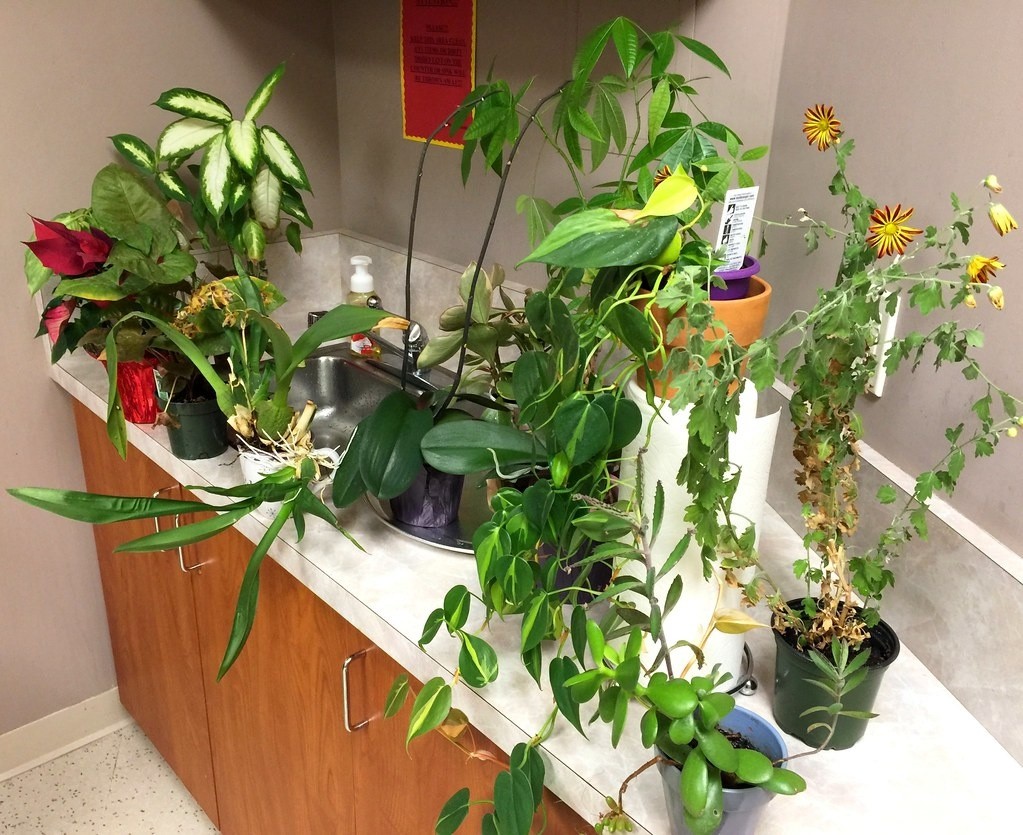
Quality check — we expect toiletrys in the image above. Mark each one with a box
[348,255,381,357]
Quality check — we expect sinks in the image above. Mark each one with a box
[225,328,458,487]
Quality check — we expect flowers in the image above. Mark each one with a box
[653,99,1023,656]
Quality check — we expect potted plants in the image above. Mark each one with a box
[7,19,805,835]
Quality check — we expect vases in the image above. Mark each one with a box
[632,276,773,403]
[767,599,901,751]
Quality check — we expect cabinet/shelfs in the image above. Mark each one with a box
[71,401,356,835]
[345,622,597,835]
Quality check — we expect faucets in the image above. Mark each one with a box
[309,296,446,391]
